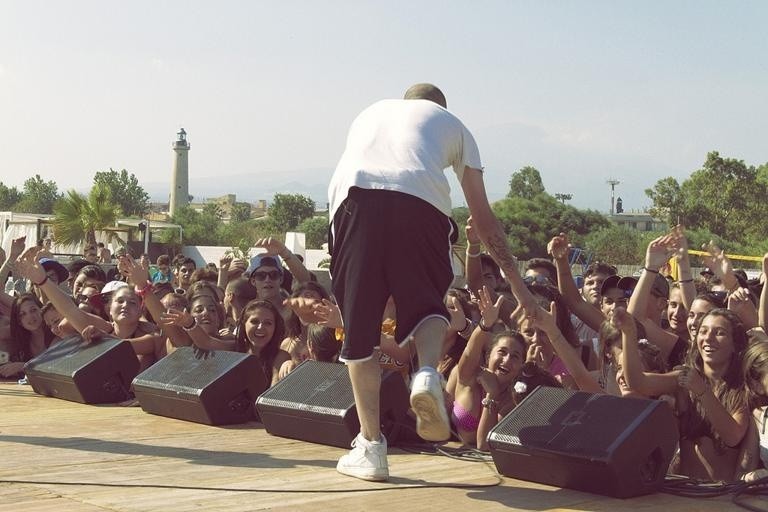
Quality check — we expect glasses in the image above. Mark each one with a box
[521,272,553,285]
[251,270,282,282]
[99,296,110,305]
[174,288,187,296]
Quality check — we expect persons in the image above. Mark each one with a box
[1,215,768,482]
[327,83,540,484]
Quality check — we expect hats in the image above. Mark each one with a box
[36,257,69,284]
[87,279,129,312]
[600,273,623,297]
[699,267,715,277]
[617,267,671,302]
[245,252,286,281]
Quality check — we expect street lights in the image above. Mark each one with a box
[606,179,620,214]
[139,218,150,257]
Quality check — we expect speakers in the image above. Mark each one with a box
[486,385,681,498]
[256,359,421,452]
[23,334,143,404]
[131,345,265,427]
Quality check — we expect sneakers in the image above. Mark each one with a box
[410,367,454,445]
[336,431,392,483]
